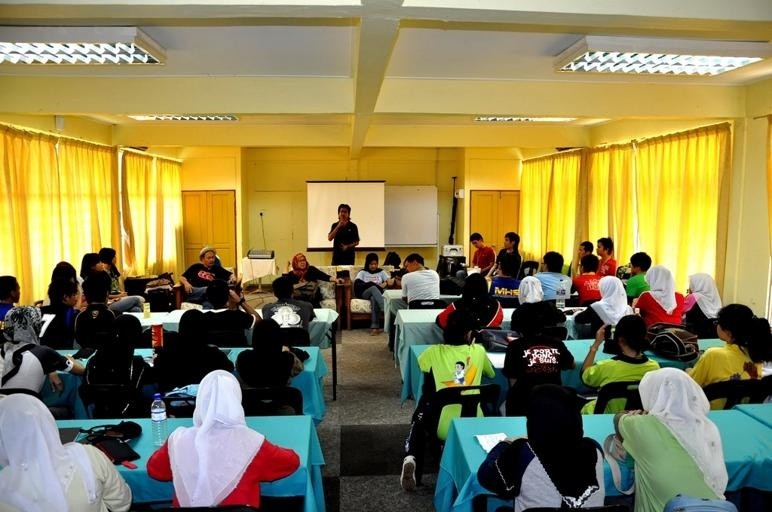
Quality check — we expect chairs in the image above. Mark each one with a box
[173,267,242,311]
[290,265,343,330]
[344,265,395,329]
[518,261,539,281]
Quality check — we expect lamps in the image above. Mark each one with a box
[1,25,167,67]
[553,36,766,79]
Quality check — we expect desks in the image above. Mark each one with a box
[243,257,275,293]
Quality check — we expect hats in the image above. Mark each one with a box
[200,247,215,260]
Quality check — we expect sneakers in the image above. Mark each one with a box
[400,455,416,490]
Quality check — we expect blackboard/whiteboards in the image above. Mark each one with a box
[384,184,440,247]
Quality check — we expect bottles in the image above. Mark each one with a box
[150,392,168,446]
[556,279,566,310]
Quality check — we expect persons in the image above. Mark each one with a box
[353,232,772,512]
[329,203,360,266]
[1,248,337,511]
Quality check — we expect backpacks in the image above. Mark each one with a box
[648,322,698,361]
